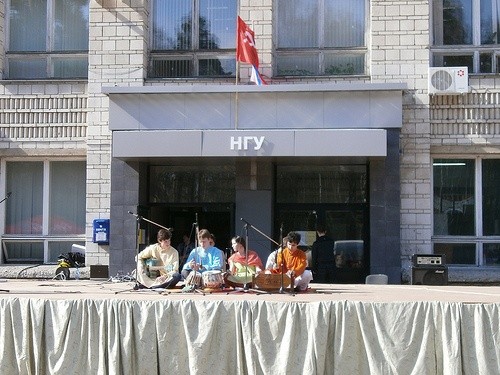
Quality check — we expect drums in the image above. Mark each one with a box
[202,270,223,287]
[254,273,291,288]
[186,270,202,287]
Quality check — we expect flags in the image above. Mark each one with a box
[236,16,269,87]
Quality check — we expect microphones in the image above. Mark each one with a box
[169,227,174,232]
[0,191,12,203]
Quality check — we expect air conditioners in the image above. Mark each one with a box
[428,66,471,95]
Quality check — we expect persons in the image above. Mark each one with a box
[208,234,226,274]
[269,233,311,292]
[325,243,359,284]
[312,222,334,284]
[265,236,288,275]
[176,233,195,274]
[181,230,224,288]
[130,229,181,290]
[224,234,264,289]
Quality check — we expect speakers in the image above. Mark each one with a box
[412,265,448,286]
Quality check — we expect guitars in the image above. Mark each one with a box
[144,258,173,278]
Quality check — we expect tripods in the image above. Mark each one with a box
[114,210,298,297]
